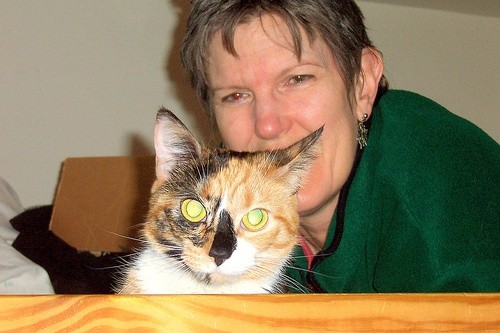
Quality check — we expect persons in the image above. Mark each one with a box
[179,1,499,295]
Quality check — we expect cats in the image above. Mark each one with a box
[110,107,326,295]
